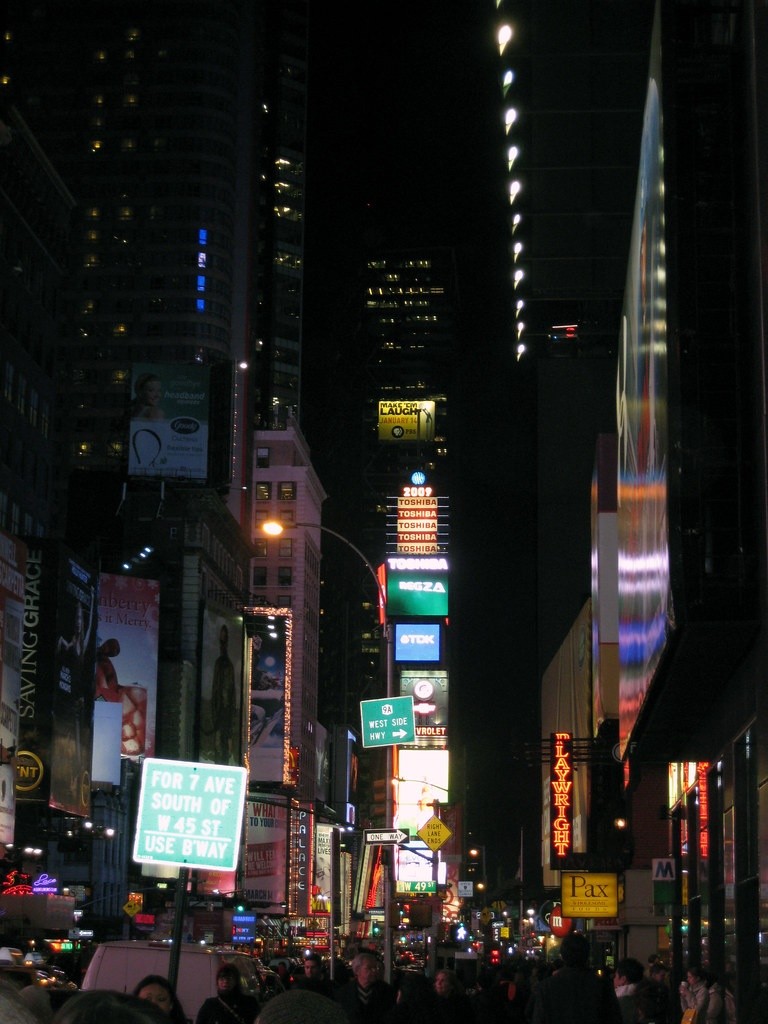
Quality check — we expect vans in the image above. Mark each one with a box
[82,942,279,1014]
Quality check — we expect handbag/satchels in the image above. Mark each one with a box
[682,1008,699,1024]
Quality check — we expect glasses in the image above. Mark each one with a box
[219,975,234,981]
[360,966,378,972]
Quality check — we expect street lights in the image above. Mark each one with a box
[397,776,467,883]
[263,521,394,989]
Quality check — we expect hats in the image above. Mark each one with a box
[258,990,348,1024]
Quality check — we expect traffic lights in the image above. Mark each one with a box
[450,925,471,947]
[372,923,379,937]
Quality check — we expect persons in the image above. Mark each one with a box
[133,974,187,1024]
[388,969,475,1024]
[132,373,164,418]
[211,625,236,764]
[52,989,170,1024]
[334,953,398,1024]
[292,954,334,1001]
[724,976,737,1024]
[515,933,623,1024]
[614,954,669,1024]
[678,968,723,1024]
[194,964,258,1024]
[0,975,52,1024]
[278,962,289,990]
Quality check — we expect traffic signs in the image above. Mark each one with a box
[360,695,419,750]
[362,829,410,845]
